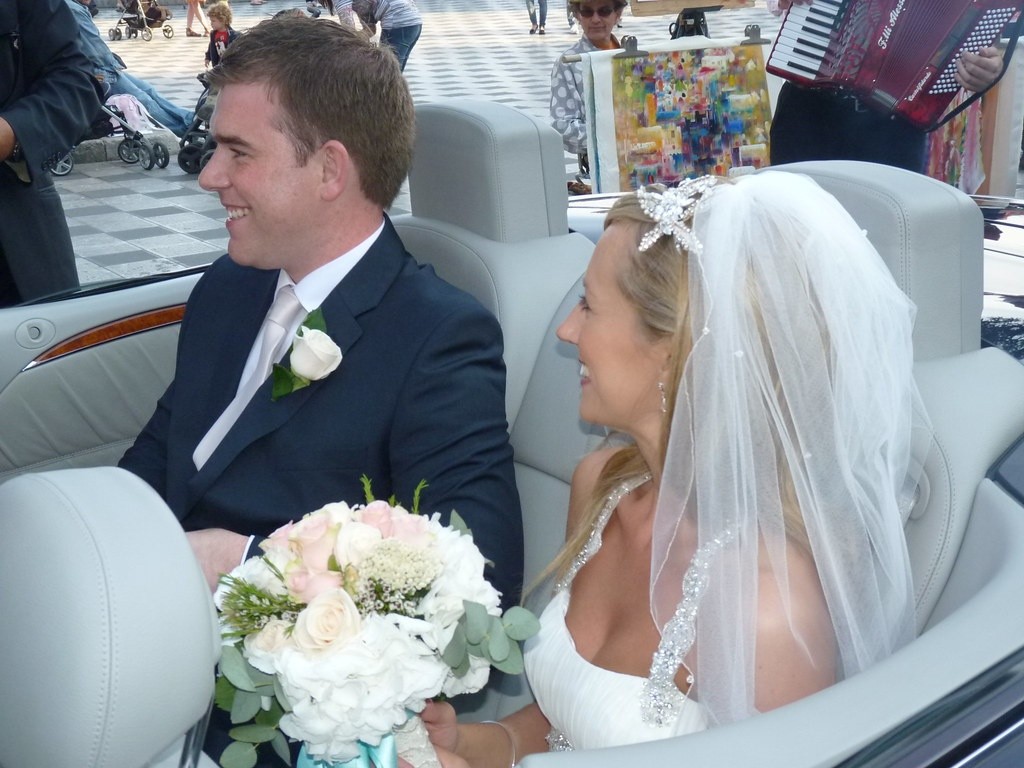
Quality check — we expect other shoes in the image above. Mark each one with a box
[186,28,200,36]
[539,25,544,34]
[530,25,538,34]
[204,31,209,37]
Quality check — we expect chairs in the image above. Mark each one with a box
[509,156,983,637]
[388,92,595,422]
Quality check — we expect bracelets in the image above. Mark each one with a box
[480,721,516,768]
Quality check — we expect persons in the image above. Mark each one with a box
[274,8,305,18]
[77,0,98,17]
[370,171,935,768]
[551,0,627,175]
[617,17,623,27]
[205,4,244,68]
[116,16,525,768]
[305,0,321,18]
[567,0,578,33]
[318,0,423,75]
[526,0,547,34]
[767,0,1004,174]
[0,0,104,309]
[185,0,210,36]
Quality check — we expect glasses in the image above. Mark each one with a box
[577,7,616,17]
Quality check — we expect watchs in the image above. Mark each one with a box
[7,140,24,163]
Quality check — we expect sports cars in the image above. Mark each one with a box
[0,95,1024,767]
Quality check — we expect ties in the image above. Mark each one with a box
[193,286,302,473]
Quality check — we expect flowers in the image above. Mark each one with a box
[214,475,541,768]
[268,309,344,400]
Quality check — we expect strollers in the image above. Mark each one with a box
[107,0,175,41]
[49,79,168,176]
[179,73,218,173]
